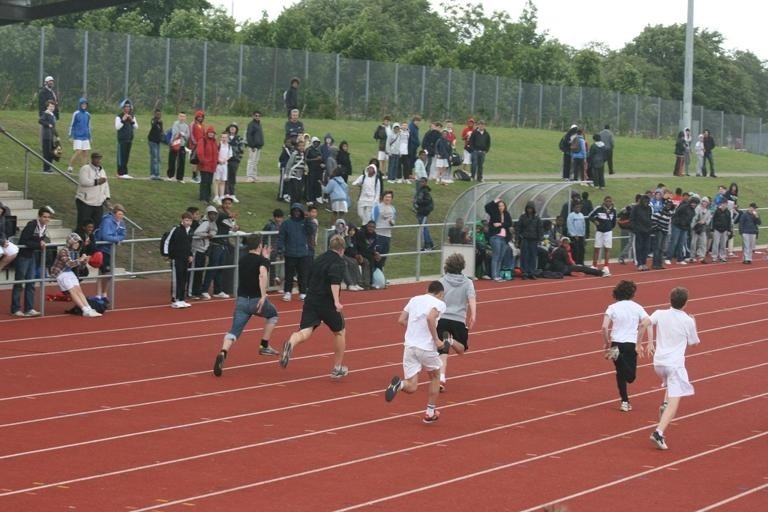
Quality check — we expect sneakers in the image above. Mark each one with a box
[382,175,484,186]
[618,251,752,272]
[168,277,391,308]
[283,195,329,208]
[563,176,606,190]
[605,346,667,452]
[42,166,261,183]
[201,194,240,206]
[13,296,111,317]
[214,332,454,423]
[673,173,718,178]
[473,264,611,281]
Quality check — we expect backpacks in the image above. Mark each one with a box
[570,137,581,153]
[558,133,570,151]
[617,205,635,230]
[160,231,170,256]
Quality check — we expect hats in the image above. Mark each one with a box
[45,76,55,82]
[206,205,218,214]
[432,118,486,133]
[750,202,758,208]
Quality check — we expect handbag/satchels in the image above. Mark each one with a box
[372,268,385,290]
[450,149,462,166]
[348,194,353,209]
[453,169,470,181]
[160,128,173,145]
[466,143,473,153]
[88,251,103,268]
[49,135,64,162]
[190,144,198,164]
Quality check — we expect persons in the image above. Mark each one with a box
[383,282,445,424]
[213,233,280,376]
[375,114,491,185]
[38,75,383,224]
[558,123,616,189]
[281,235,348,379]
[439,253,477,392]
[412,177,435,251]
[599,280,656,413]
[673,127,716,177]
[635,287,701,450]
[449,182,762,283]
[2,153,128,318]
[160,190,396,308]
